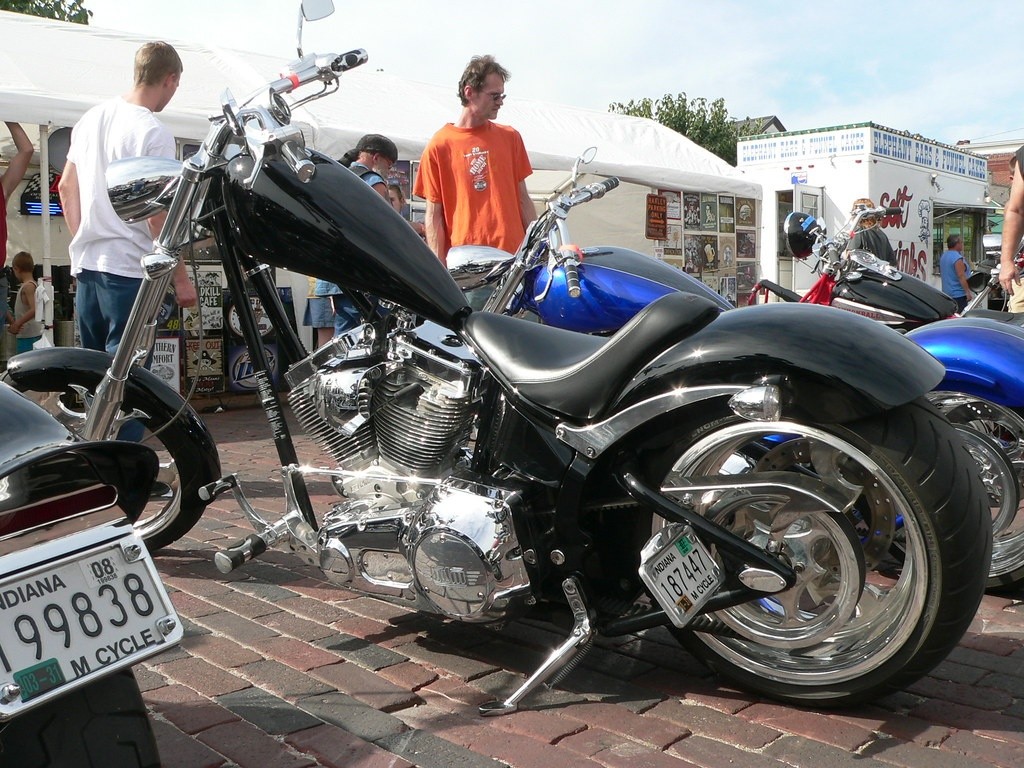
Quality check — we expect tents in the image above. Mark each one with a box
[0,8,764,349]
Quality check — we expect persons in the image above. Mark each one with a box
[846,199,898,270]
[0,41,425,498]
[998,146,1024,313]
[412,55,539,311]
[939,234,973,315]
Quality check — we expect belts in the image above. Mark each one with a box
[0,265,13,279]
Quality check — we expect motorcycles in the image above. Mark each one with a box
[1,0,1022,768]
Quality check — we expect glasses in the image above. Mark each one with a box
[480,87,507,101]
[382,155,393,170]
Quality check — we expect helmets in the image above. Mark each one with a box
[355,134,399,164]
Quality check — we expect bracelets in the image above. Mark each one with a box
[420,222,425,238]
